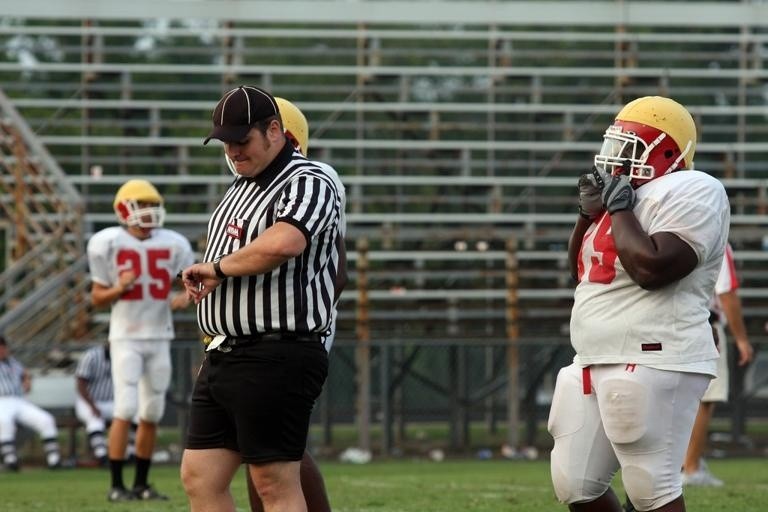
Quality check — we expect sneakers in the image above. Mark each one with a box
[102,479,167,503]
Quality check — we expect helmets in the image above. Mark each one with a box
[267,91,316,161]
[597,91,699,185]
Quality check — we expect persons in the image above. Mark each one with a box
[681,241,755,489]
[181,84,342,511]
[86,179,195,500]
[223,96,350,511]
[1,337,75,472]
[547,97,734,512]
[74,340,140,465]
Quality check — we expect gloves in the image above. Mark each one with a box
[592,163,635,218]
[571,166,606,220]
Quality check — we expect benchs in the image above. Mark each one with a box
[18,407,179,468]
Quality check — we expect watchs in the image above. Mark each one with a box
[212,252,231,280]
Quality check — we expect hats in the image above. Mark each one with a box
[197,83,277,149]
[113,179,167,234]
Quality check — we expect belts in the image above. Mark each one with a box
[219,327,324,349]
